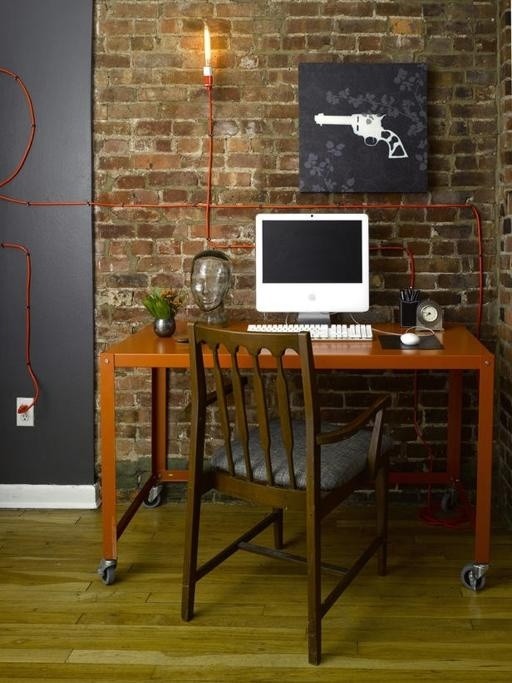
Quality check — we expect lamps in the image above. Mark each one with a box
[199,20,216,89]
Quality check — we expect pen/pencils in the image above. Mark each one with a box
[400,288,420,302]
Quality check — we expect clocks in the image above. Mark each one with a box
[414,298,445,332]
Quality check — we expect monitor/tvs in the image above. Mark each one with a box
[256,213,370,323]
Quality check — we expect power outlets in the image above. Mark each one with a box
[13,396,34,428]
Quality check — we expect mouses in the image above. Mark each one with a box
[400,333,420,346]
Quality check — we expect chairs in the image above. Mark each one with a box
[171,314,398,666]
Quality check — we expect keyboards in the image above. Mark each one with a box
[247,324,373,341]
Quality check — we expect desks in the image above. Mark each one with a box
[95,303,497,599]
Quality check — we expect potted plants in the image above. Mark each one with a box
[143,285,186,339]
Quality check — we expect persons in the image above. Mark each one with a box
[189,249,234,325]
